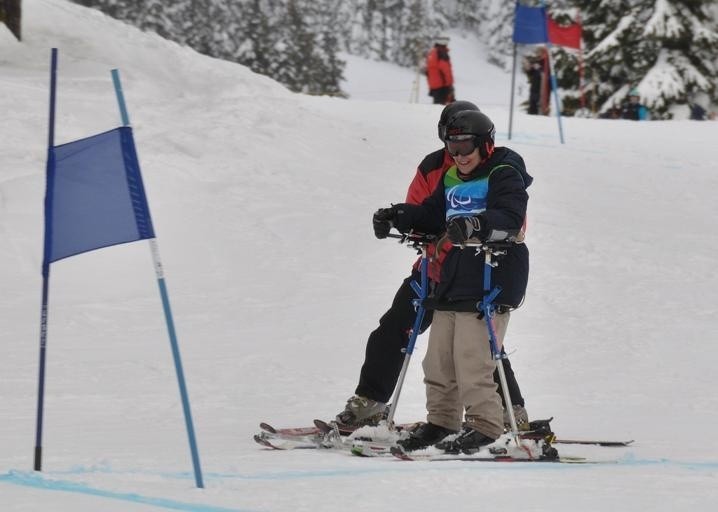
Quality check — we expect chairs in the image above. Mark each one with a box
[335,392,530,450]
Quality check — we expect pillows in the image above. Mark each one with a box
[438,101,496,159]
[435,30,450,48]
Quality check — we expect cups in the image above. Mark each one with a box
[446,127,496,157]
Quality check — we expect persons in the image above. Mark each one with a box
[337,101,529,432]
[427,36,456,106]
[373,110,534,454]
[523,45,552,116]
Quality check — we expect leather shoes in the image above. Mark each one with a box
[373,207,397,238]
[446,214,492,242]
